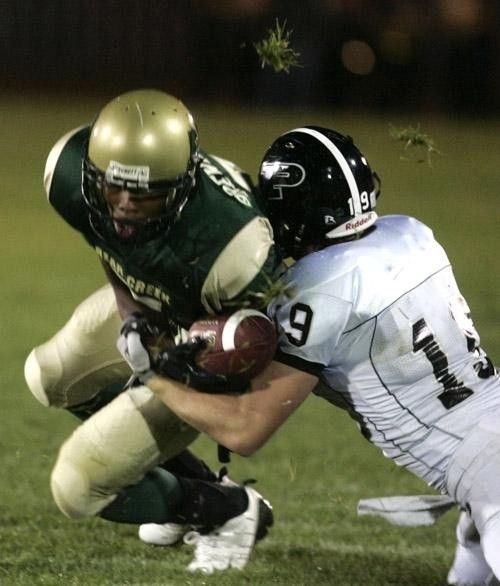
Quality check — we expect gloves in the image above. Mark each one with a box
[116,312,225,394]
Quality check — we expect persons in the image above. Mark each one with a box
[24,89,290,574]
[117,126,500,585]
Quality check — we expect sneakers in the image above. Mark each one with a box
[139,472,240,546]
[189,485,274,573]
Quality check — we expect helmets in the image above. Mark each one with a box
[259,126,381,258]
[82,89,199,247]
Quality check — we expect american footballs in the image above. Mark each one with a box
[173,308,281,382]
